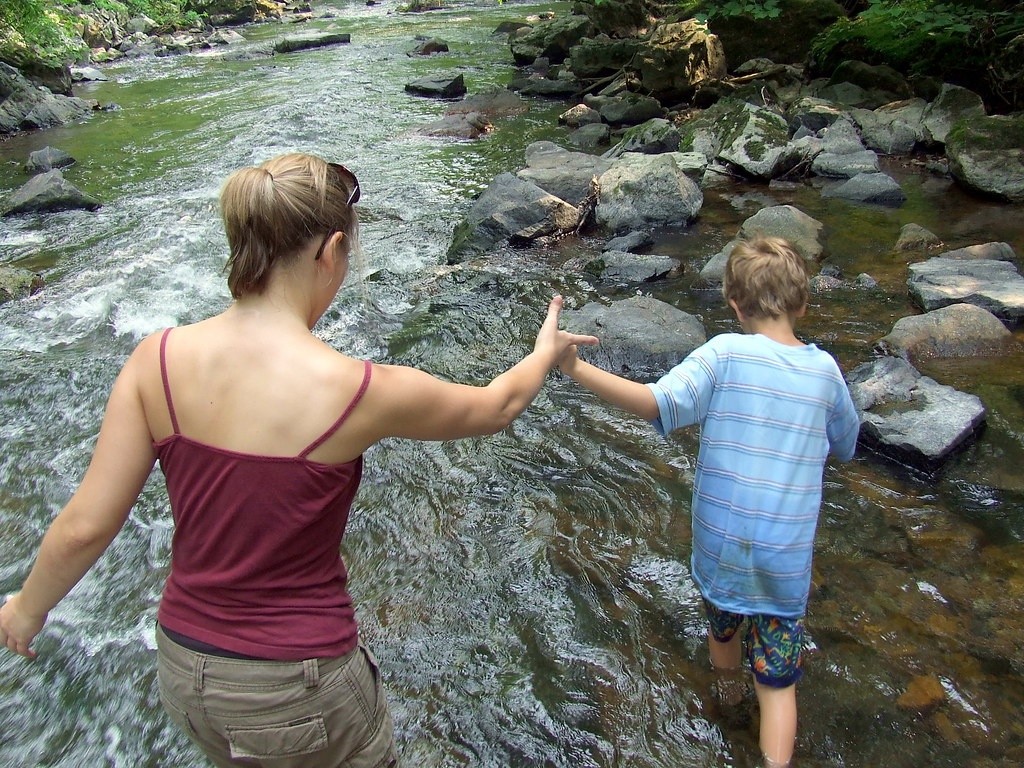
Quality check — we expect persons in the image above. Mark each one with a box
[1,154,598,768]
[555,235,858,768]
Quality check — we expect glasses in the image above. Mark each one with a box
[314,163,360,260]
